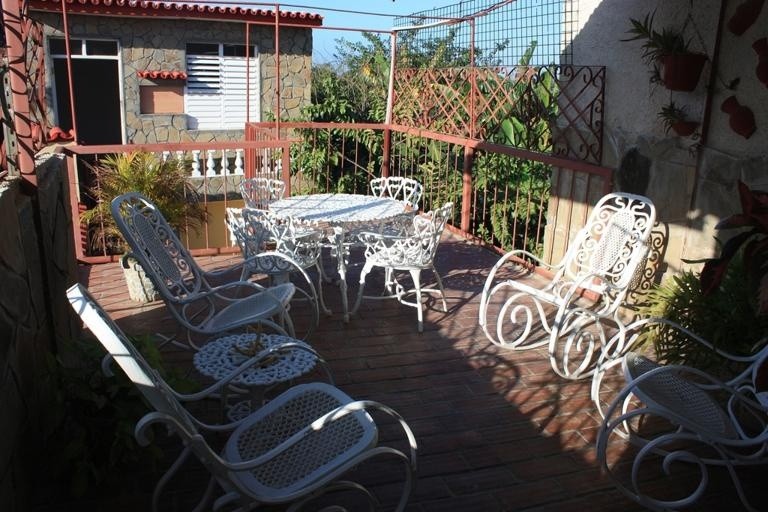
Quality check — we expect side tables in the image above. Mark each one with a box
[191,331,320,429]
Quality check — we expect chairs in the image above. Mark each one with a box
[224,206,333,325]
[370,176,426,208]
[108,191,321,352]
[589,316,768,510]
[352,200,457,334]
[477,190,658,382]
[64,278,422,511]
[238,177,287,211]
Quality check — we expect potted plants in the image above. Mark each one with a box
[655,100,703,141]
[617,3,709,94]
[78,146,214,304]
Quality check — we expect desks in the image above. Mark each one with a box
[267,192,420,322]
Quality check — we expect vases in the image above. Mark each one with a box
[727,0,765,36]
[751,37,768,90]
[720,95,758,140]
